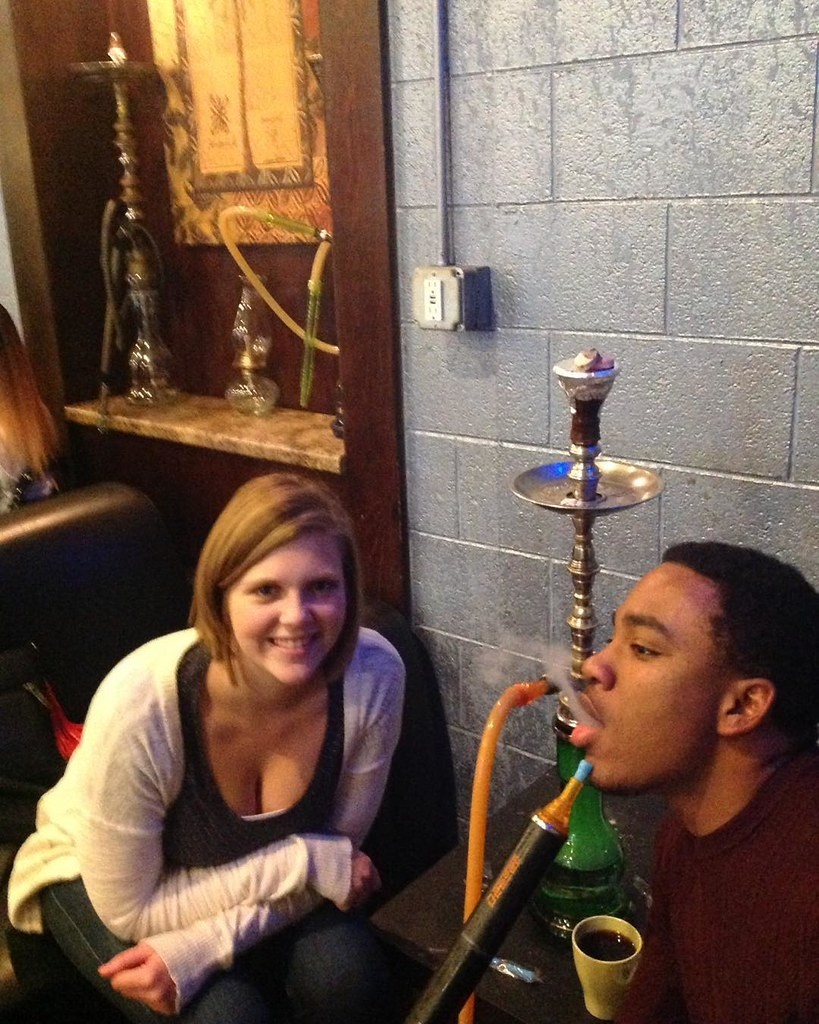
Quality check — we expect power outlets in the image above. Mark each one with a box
[410,267,475,332]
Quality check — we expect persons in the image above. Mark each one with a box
[0,302,103,513]
[570,541,819,1023]
[6,472,406,1023]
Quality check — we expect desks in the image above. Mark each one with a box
[369,766,670,1024]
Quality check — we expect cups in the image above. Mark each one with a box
[572,914,643,1020]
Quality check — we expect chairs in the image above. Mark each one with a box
[1,481,178,821]
[8,594,460,1024]
